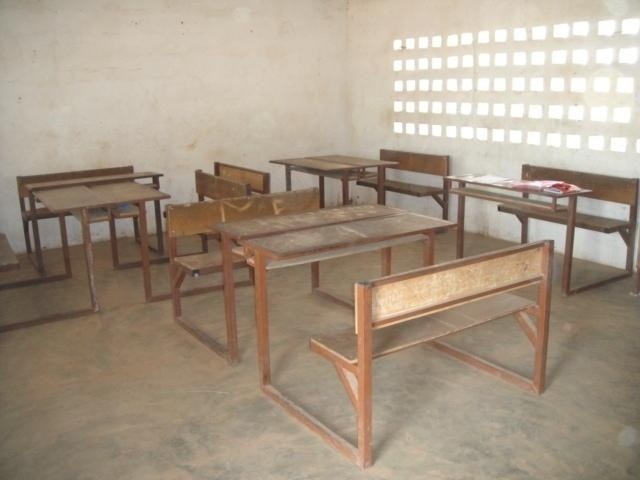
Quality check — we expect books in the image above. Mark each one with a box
[513,180,581,194]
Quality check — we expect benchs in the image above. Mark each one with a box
[1,150,639,469]
